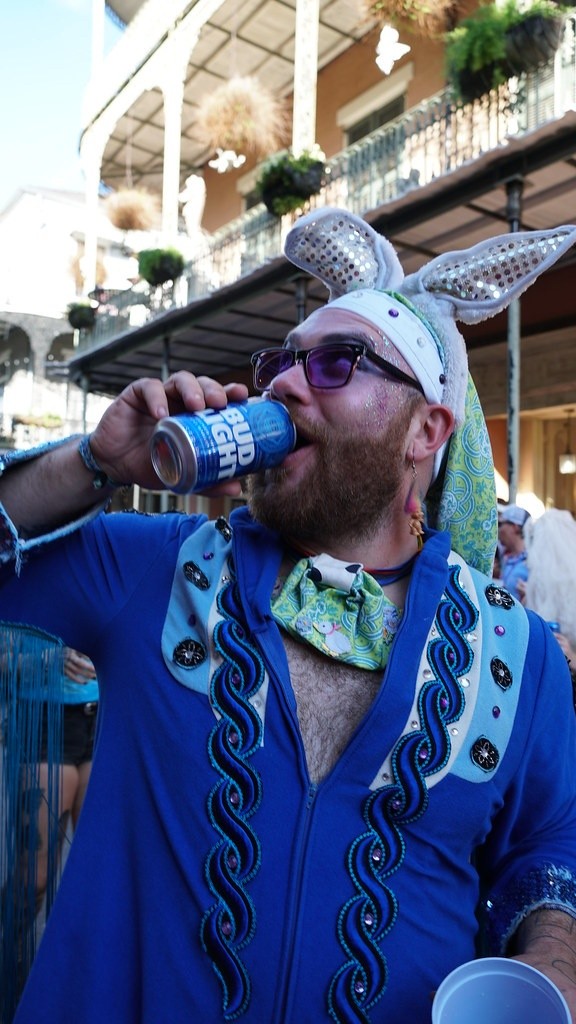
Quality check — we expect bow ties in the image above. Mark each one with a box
[269,536,420,673]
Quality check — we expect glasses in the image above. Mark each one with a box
[250,342,424,391]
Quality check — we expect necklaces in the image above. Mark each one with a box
[281,535,426,588]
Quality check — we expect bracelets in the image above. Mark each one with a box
[78,433,132,492]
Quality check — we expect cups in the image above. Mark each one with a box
[433,955,571,1024]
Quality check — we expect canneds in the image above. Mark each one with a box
[148,396,298,494]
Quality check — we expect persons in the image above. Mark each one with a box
[495,497,576,686]
[0,629,98,983]
[2,287,576,1023]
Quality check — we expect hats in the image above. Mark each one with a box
[498,508,531,526]
